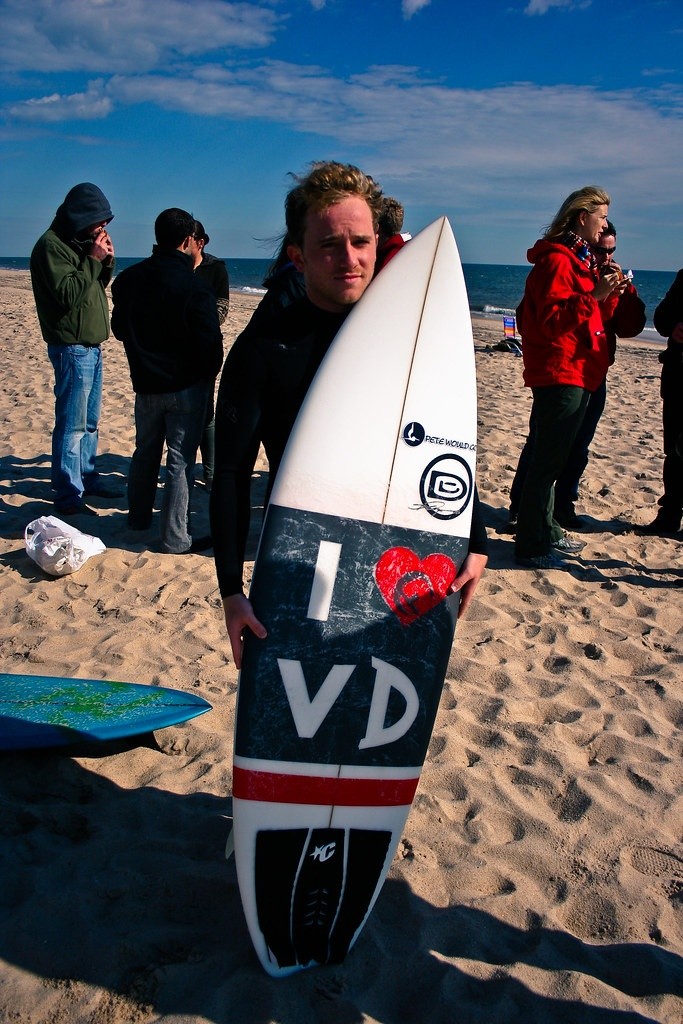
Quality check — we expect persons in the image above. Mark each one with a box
[207,163,489,671]
[646,268,683,536]
[30,183,124,516]
[111,207,229,554]
[506,186,646,570]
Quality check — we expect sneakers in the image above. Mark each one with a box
[204,476,213,492]
[525,531,589,569]
[506,511,521,528]
[85,482,124,499]
[552,502,584,529]
[55,500,98,517]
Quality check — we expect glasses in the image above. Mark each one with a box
[590,246,616,255]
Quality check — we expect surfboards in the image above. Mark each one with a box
[226,215,483,984]
[0,671,213,757]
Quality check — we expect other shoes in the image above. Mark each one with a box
[176,535,213,554]
[637,506,682,536]
